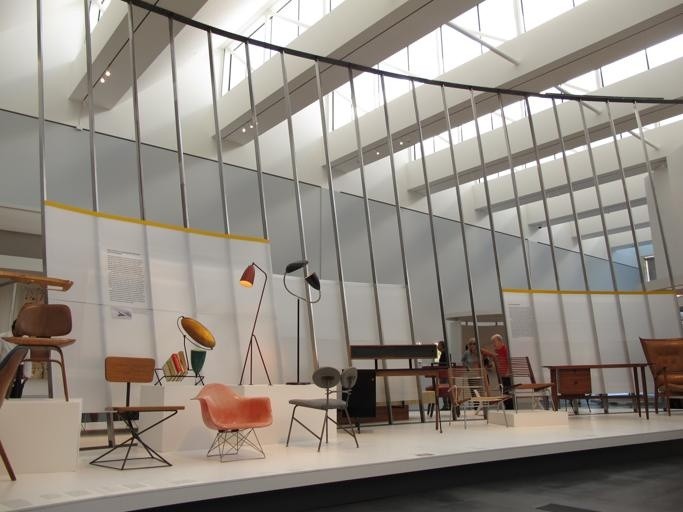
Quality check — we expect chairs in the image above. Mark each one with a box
[638,337,683,416]
[492,357,557,413]
[422,365,464,417]
[90,356,185,470]
[286,367,359,452]
[191,383,273,463]
[1,304,76,402]
[447,368,511,430]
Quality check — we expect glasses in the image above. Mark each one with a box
[469,343,476,345]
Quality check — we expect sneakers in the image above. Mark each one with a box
[440,406,450,411]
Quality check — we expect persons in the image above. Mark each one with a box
[461,338,485,410]
[482,334,514,410]
[0,320,32,398]
[437,341,453,411]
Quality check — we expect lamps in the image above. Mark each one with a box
[284,260,321,385]
[239,262,272,385]
[191,350,207,385]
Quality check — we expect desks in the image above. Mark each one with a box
[541,362,656,421]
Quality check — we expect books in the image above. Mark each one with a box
[162,351,188,381]
